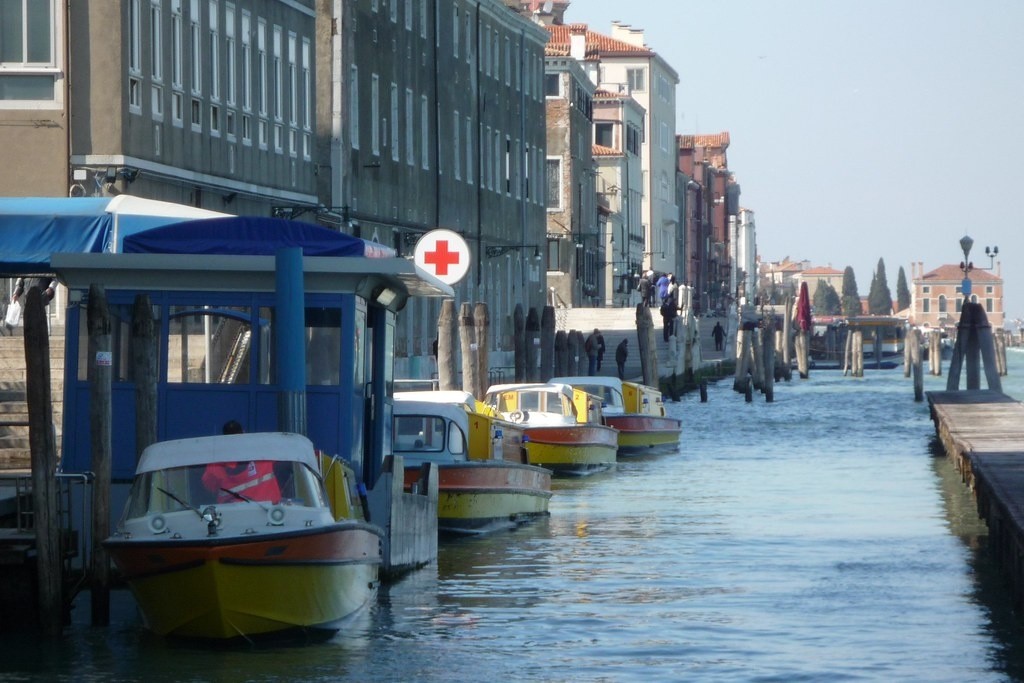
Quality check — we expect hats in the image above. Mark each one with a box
[593,328,601,334]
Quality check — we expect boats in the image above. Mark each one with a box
[549,376,682,456]
[59,216,437,641]
[393,387,555,539]
[1,188,239,623]
[483,382,622,474]
[809,315,911,359]
[914,324,953,360]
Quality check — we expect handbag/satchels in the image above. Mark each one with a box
[637,283,641,291]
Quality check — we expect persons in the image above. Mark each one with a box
[597,335,605,371]
[201,420,281,505]
[616,339,628,380]
[13,277,58,306]
[711,322,726,351]
[585,328,601,376]
[638,272,683,343]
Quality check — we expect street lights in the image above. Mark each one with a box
[975,246,999,270]
[958,233,973,305]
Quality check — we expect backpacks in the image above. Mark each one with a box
[585,335,592,352]
